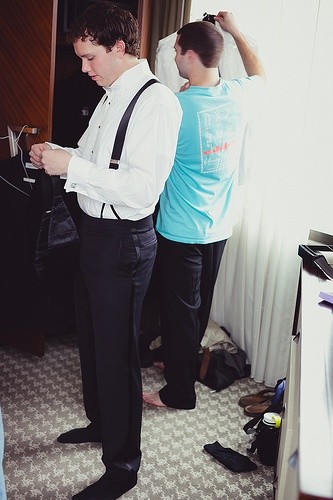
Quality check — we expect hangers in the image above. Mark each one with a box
[0,125,33,197]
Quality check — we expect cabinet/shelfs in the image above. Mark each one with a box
[1,0,154,164]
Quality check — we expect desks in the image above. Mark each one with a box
[272,251,333,500]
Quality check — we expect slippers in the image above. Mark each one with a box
[239,387,275,407]
[244,395,284,416]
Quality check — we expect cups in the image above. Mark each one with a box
[258,412,282,467]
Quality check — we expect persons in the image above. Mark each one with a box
[143,11,265,410]
[28,3,183,500]
